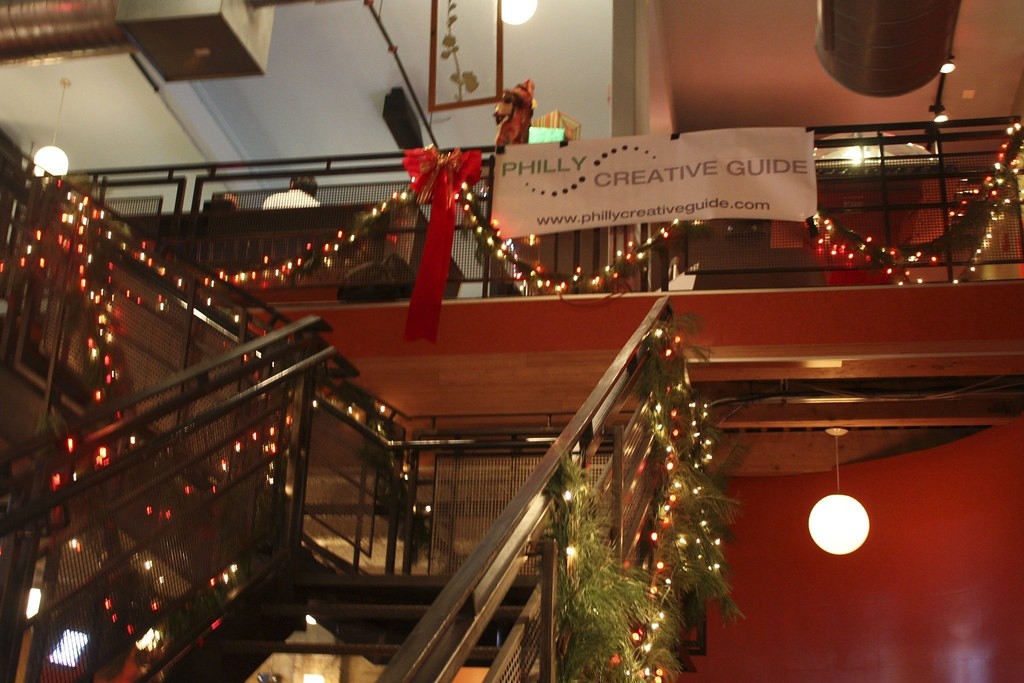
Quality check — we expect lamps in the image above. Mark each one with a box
[33,78,69,178]
[807,427,869,554]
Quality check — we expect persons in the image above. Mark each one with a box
[262,174,321,210]
[214,194,240,211]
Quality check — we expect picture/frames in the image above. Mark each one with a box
[428,0,504,110]
